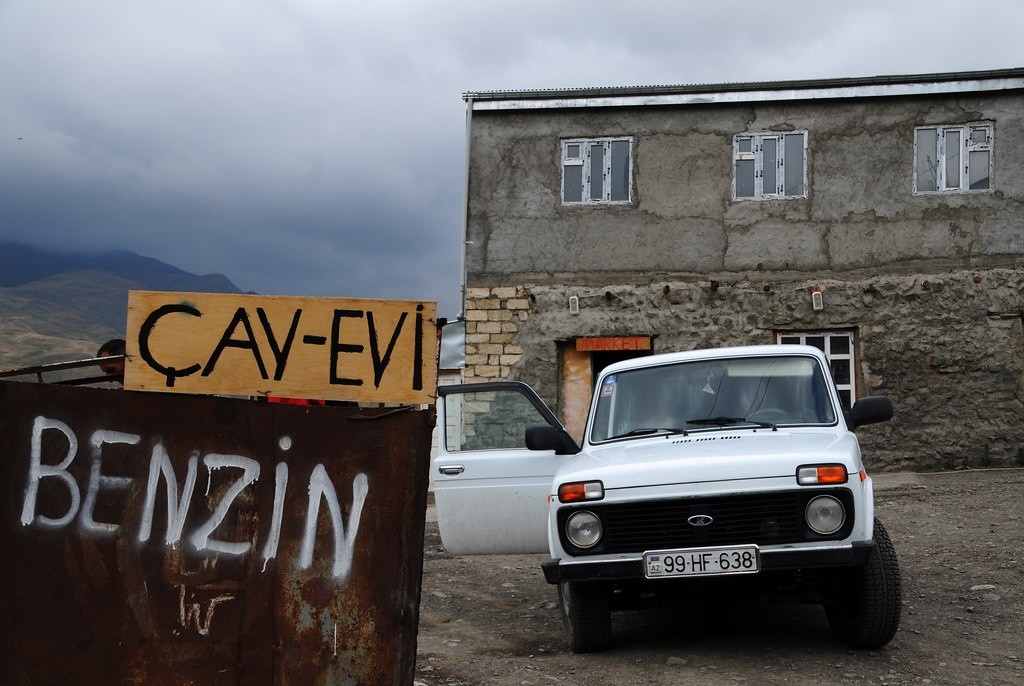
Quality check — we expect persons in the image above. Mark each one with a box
[94,336,128,384]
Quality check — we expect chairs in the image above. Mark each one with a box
[646,396,679,424]
[736,378,779,419]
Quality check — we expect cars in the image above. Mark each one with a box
[432,338,904,655]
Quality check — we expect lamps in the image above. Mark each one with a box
[812,292,823,311]
[570,296,579,314]
